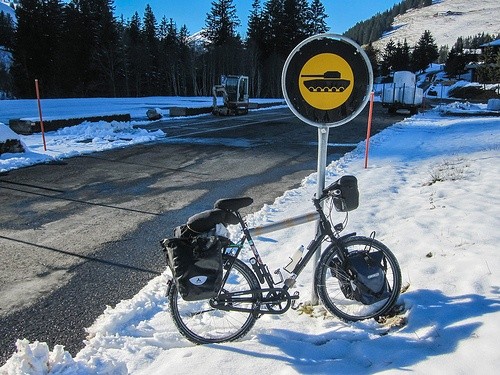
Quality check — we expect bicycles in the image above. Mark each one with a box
[160,175,405,347]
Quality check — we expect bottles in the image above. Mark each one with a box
[284,244,305,273]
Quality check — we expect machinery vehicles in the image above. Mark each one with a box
[212,72,250,118]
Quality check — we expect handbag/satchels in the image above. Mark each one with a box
[163,238,222,301]
[175,210,240,239]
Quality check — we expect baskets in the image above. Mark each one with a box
[330,175,358,212]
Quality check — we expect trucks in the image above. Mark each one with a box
[378,82,424,116]
